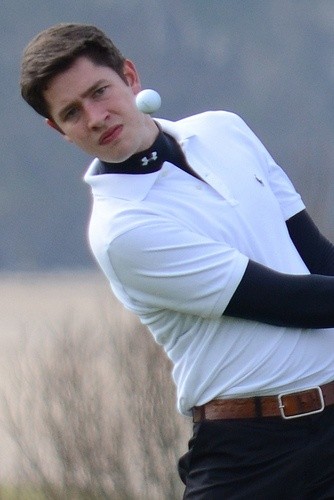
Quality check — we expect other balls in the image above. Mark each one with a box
[135,87,161,114]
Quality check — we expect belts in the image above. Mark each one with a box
[191,380,334,423]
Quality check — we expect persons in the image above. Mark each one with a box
[15,20,334,500]
